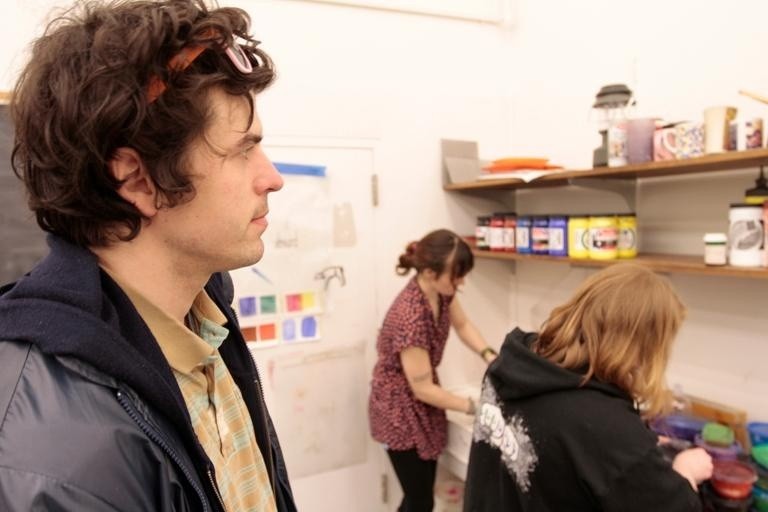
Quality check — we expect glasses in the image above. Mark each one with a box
[140,25,255,106]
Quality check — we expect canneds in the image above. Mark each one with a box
[475,211,638,261]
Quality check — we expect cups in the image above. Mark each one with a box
[607,106,768,170]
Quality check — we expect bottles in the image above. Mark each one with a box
[726,165,768,270]
[476,211,638,264]
[704,231,727,267]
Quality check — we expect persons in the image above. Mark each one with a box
[1,0,299,510]
[462,260,717,511]
[367,227,496,511]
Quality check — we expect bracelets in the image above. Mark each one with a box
[465,396,476,416]
[481,347,495,362]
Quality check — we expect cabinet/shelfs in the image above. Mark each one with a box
[443,147,768,282]
